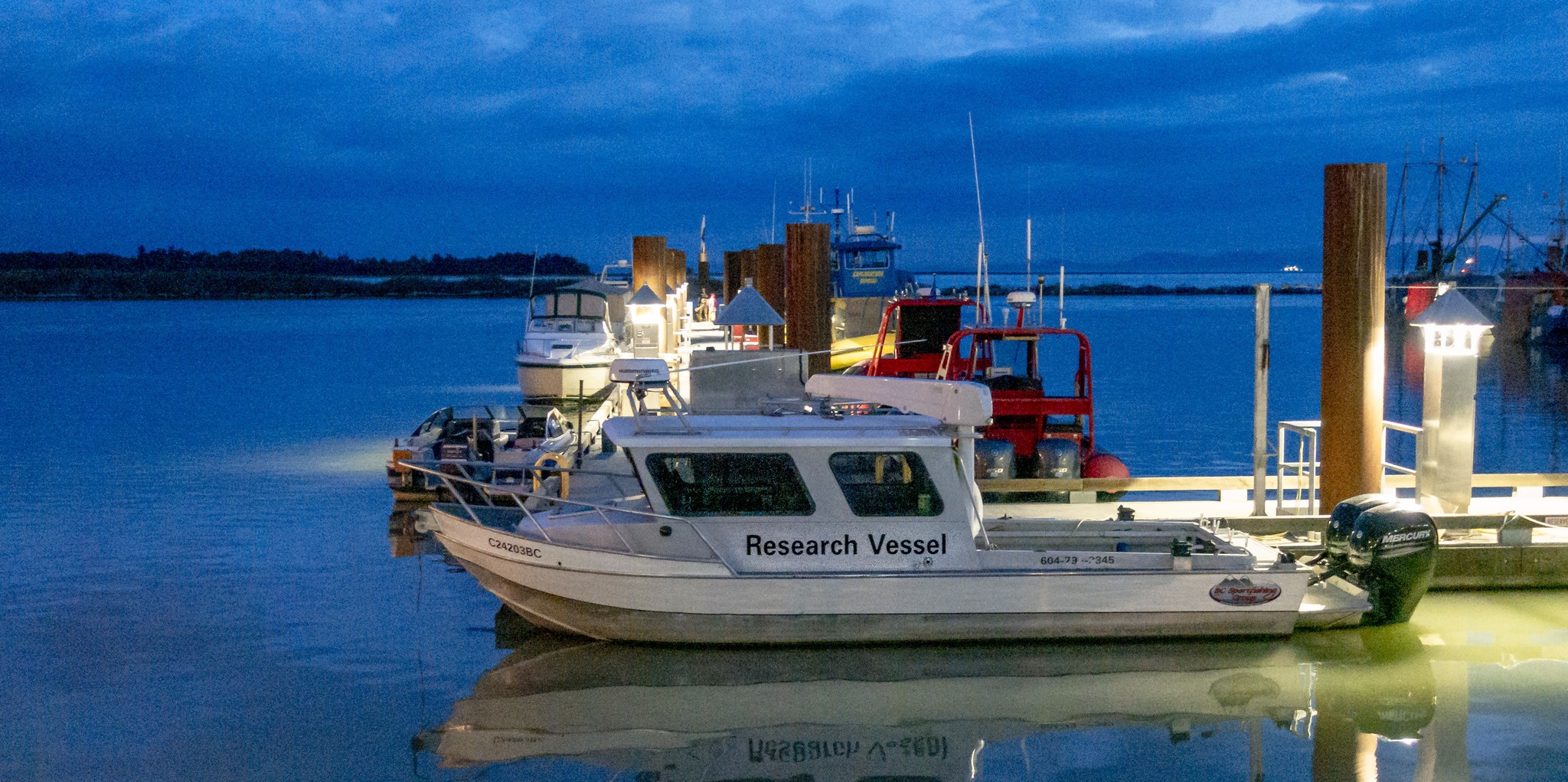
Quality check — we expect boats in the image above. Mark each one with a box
[1380,132,1511,349]
[514,108,1133,494]
[411,624,1436,775]
[402,273,1447,645]
